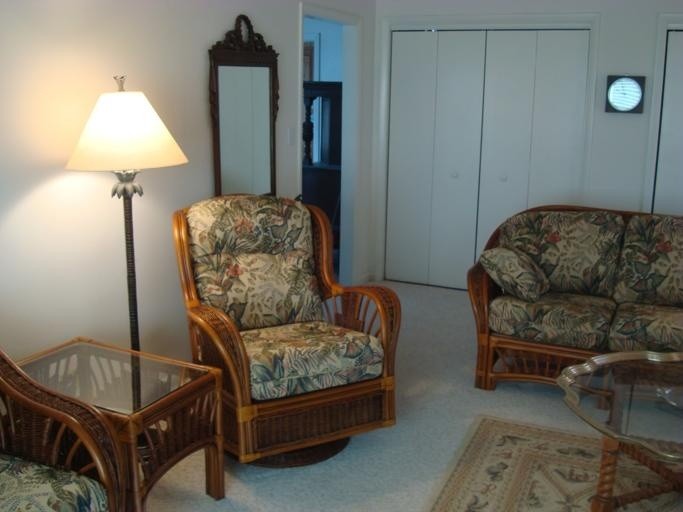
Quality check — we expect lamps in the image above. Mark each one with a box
[59,73,189,355]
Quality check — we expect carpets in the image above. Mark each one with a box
[422,413,683,512]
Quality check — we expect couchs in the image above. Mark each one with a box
[0,346,130,512]
[462,201,682,414]
[170,192,404,472]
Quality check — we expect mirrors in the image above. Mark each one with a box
[204,11,283,200]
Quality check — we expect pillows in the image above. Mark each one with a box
[476,245,551,304]
